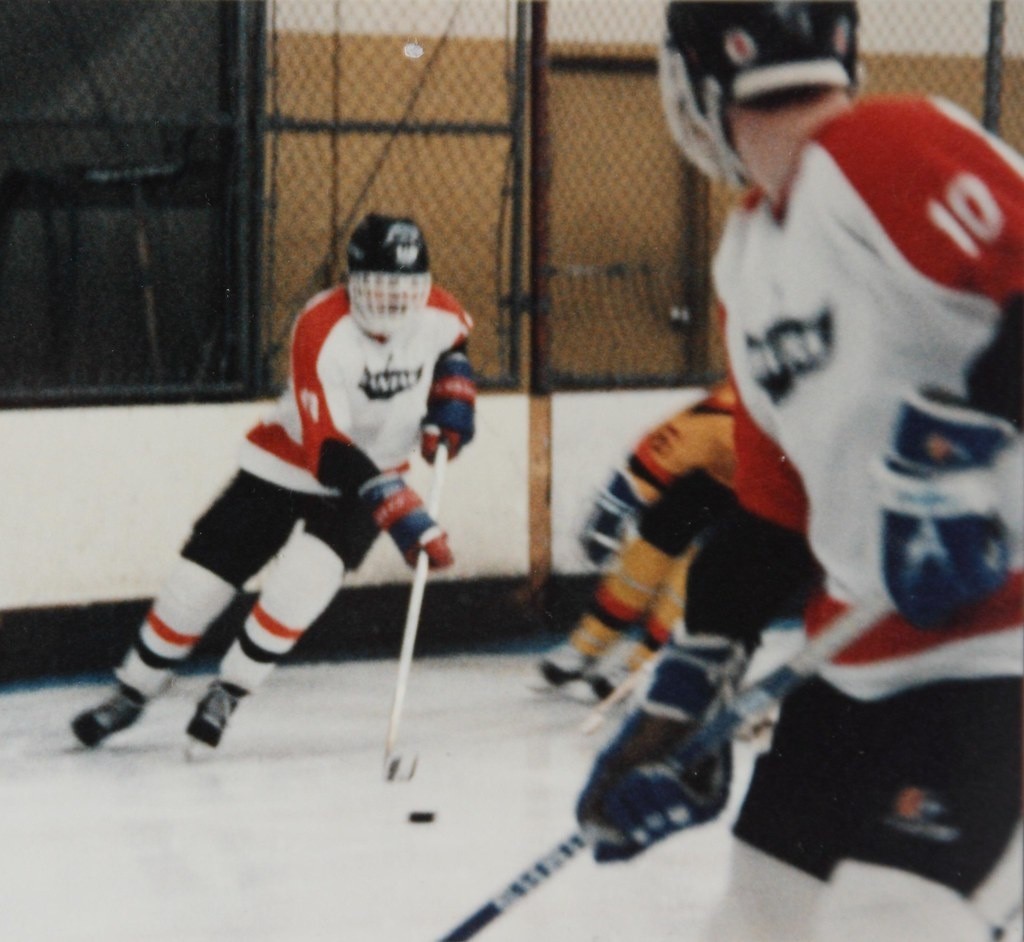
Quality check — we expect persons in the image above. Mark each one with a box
[576,0,1024,942]
[72,212,475,748]
[537,383,737,704]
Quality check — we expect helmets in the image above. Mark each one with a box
[664,0,864,99]
[347,210,434,277]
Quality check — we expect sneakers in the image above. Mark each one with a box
[184,684,246,757]
[526,646,585,691]
[567,665,618,701]
[68,687,143,751]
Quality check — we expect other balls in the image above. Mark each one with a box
[409,811,434,823]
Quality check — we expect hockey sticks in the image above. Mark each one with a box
[379,439,449,786]
[429,585,901,941]
[561,644,673,740]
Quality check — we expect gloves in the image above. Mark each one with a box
[879,403,1009,628]
[422,353,473,463]
[358,473,452,570]
[579,478,636,562]
[578,657,743,857]
